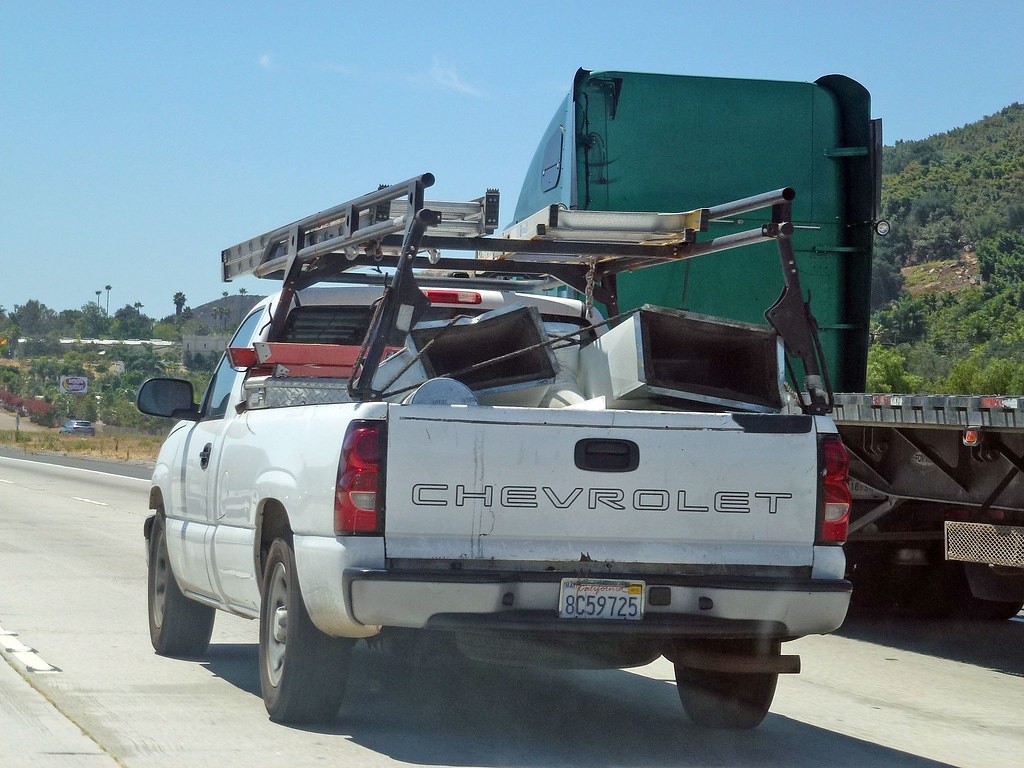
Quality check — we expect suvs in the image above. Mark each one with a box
[59,419,95,438]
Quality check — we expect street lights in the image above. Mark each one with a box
[105,285,113,318]
[95,289,102,310]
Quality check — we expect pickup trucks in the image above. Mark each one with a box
[135,277,854,735]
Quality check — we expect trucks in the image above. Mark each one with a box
[494,65,1024,628]
